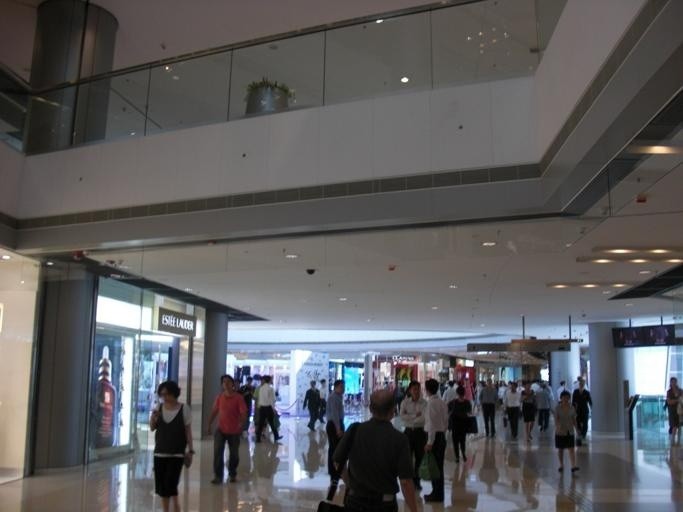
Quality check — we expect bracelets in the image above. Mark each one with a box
[188,449,195,454]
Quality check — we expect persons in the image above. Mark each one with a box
[380,380,404,416]
[442,377,593,472]
[666,377,683,446]
[423,380,450,500]
[227,428,340,507]
[401,381,427,492]
[236,373,328,442]
[449,438,583,512]
[147,381,194,512]
[203,374,248,485]
[665,448,682,512]
[333,390,418,512]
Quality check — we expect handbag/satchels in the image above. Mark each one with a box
[319,423,359,511]
[465,416,478,433]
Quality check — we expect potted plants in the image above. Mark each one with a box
[244,76,297,116]
